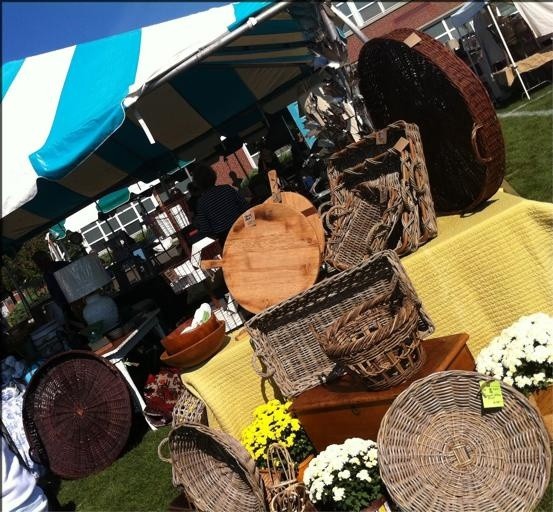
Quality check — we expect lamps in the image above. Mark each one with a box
[52,250,122,337]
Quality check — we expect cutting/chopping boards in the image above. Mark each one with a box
[261,170,326,265]
[200,203,321,315]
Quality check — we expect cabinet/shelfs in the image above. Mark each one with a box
[282,332,476,455]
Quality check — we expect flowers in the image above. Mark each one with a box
[236,396,382,510]
[469,309,552,399]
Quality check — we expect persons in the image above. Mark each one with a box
[31,250,74,313]
[229,171,243,187]
[255,142,280,184]
[186,182,203,215]
[289,128,310,161]
[118,231,136,251]
[89,249,106,265]
[192,164,249,248]
[107,238,130,262]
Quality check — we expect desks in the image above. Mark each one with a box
[83,307,166,432]
[180,195,551,428]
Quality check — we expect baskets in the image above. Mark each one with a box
[358,27,504,214]
[23,351,134,479]
[244,249,435,399]
[380,371,552,511]
[171,390,205,427]
[319,119,439,271]
[159,425,268,512]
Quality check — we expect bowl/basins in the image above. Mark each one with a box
[159,312,226,370]
